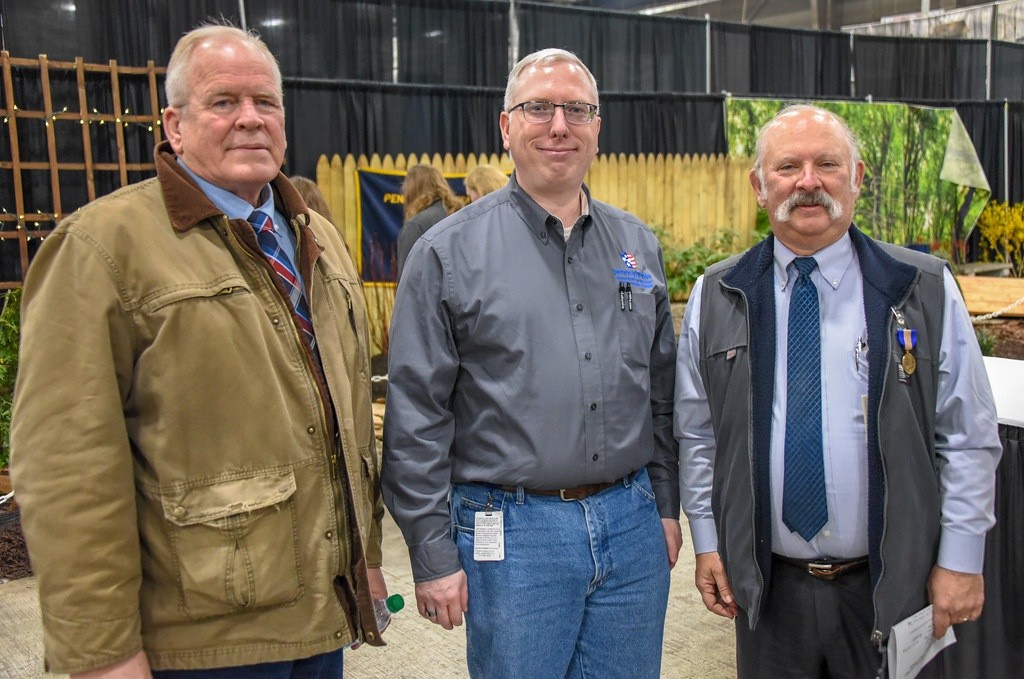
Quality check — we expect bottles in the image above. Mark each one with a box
[342,594,405,653]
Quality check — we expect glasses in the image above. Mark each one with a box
[509,100,597,125]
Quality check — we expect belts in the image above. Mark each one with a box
[491,471,638,501]
[771,551,869,580]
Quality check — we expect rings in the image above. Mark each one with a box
[427,610,436,617]
[958,615,969,621]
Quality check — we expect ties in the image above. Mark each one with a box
[246,212,323,370]
[782,257,829,542]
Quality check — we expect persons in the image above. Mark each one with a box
[394,165,510,291]
[12,25,390,679]
[380,46,682,678]
[673,103,1003,679]
